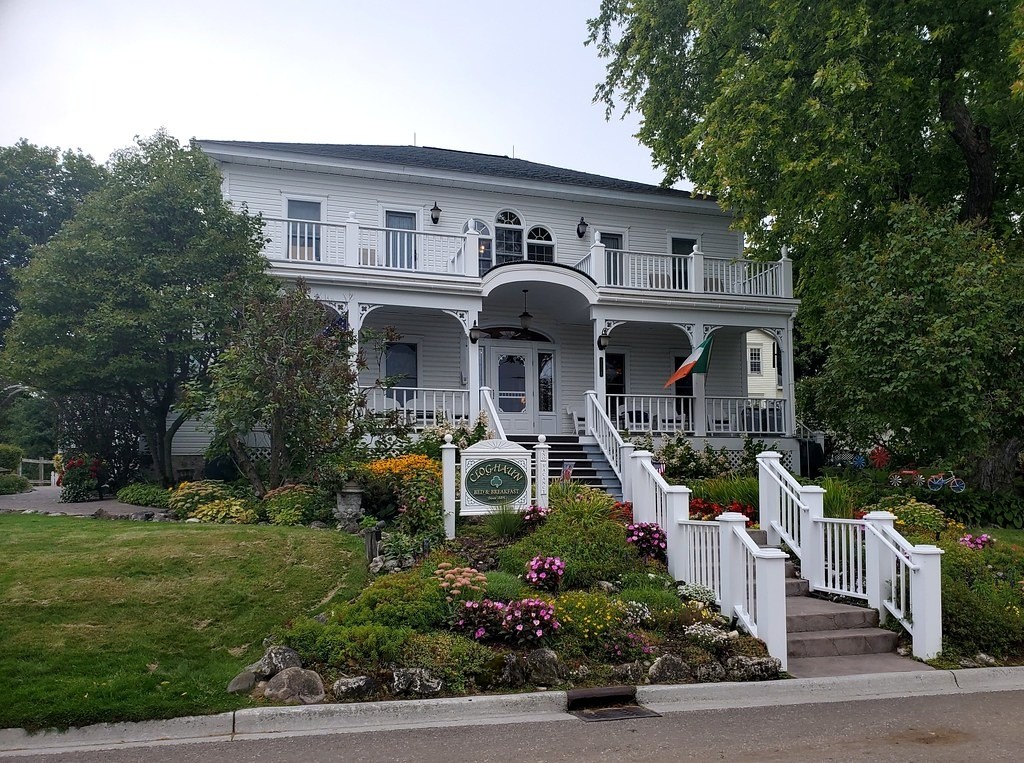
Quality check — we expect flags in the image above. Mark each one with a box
[663,335,713,389]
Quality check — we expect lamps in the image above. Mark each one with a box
[597,326,610,350]
[577,215,589,237]
[518,290,534,332]
[469,320,481,344]
[431,200,442,224]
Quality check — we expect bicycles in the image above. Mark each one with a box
[927,471,966,494]
[888,462,926,488]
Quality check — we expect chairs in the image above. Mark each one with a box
[368,396,400,425]
[706,405,735,437]
[619,402,654,431]
[407,399,437,429]
[656,403,686,431]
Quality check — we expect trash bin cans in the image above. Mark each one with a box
[741,408,781,431]
